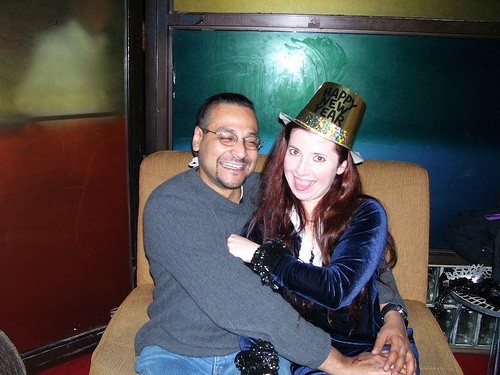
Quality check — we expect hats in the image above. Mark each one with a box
[279,82,364,163]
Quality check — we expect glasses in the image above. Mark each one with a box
[206,129,264,150]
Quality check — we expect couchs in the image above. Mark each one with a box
[89,150,465,375]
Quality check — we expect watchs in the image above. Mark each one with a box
[380,304,408,330]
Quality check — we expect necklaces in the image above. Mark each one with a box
[303,218,317,224]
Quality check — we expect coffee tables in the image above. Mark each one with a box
[449,288,500,375]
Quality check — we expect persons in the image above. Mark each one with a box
[134,93,417,375]
[226,83,421,375]
